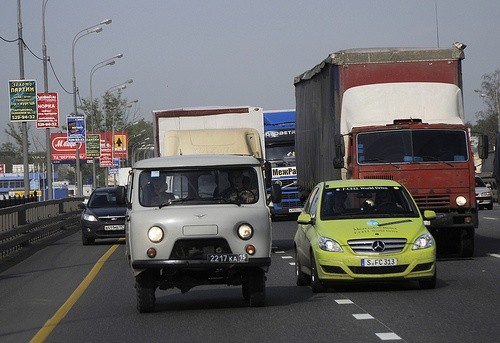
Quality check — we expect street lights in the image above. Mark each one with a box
[89,53,123,190]
[72,19,114,197]
[111,99,155,170]
[104,78,134,187]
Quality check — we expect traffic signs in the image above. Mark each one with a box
[112,131,128,159]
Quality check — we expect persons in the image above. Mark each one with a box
[220,170,250,196]
[141,175,175,205]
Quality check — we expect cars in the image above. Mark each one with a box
[77,185,128,246]
[474,175,493,210]
[294,179,437,294]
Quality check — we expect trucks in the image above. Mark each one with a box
[260,109,303,222]
[293,41,490,260]
[113,105,283,313]
